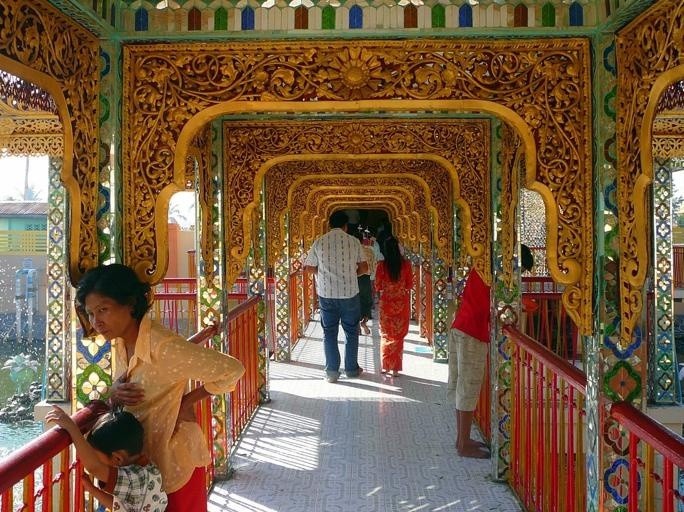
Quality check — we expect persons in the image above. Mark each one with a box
[75,263,246,512]
[347,224,389,337]
[305,210,366,383]
[445,244,535,457]
[375,236,413,377]
[43,404,167,512]
